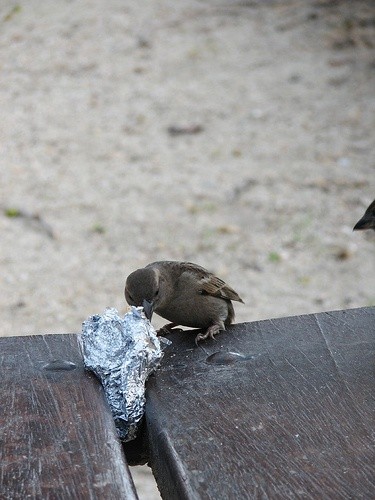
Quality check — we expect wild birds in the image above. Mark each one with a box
[352,199,374,231]
[123,259,245,347]
